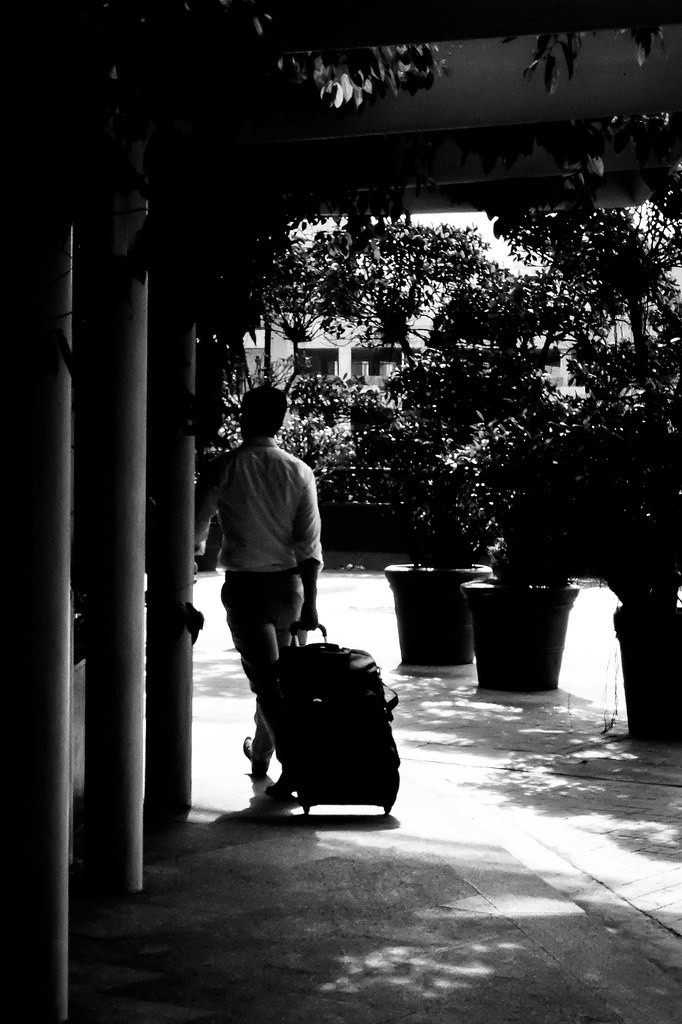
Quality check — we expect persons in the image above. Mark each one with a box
[194,385,324,776]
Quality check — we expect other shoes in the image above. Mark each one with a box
[263,769,297,796]
[244,735,267,780]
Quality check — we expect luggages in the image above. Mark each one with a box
[256,618,401,817]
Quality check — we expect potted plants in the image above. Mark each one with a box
[322,353,682,746]
